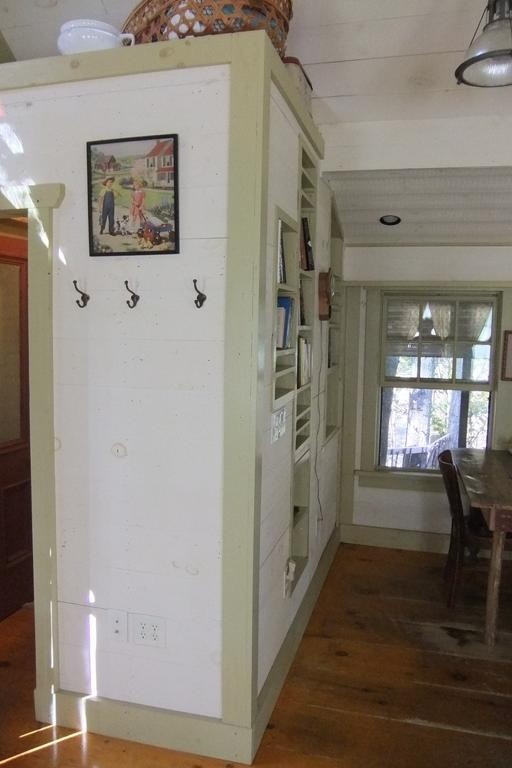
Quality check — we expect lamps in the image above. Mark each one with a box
[455,1,511,89]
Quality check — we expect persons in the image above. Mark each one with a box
[98,176,117,236]
[124,178,145,233]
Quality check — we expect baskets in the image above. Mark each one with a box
[122,0,293,61]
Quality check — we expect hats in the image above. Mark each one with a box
[102,175,115,186]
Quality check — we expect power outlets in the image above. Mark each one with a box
[150,615,167,647]
[130,612,152,643]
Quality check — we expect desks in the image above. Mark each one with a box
[450,445,510,647]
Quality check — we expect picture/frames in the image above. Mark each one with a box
[84,134,184,260]
[499,325,512,383]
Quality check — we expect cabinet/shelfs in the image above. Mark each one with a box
[257,71,341,703]
[1,215,32,622]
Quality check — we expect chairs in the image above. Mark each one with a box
[436,441,508,614]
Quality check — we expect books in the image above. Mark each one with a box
[274,213,334,390]
